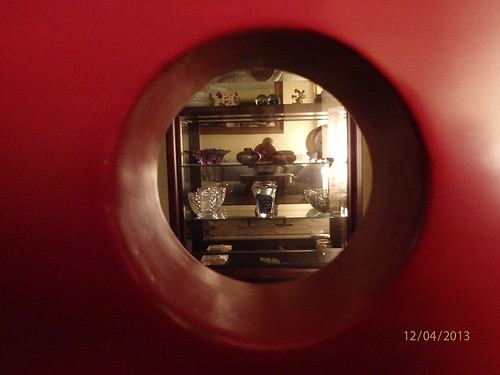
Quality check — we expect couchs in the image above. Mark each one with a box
[181,163,324,208]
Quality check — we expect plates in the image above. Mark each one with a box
[312,125,329,154]
[306,130,318,157]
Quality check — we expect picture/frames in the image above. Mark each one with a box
[182,75,283,134]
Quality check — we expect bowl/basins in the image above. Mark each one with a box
[185,150,233,165]
[196,187,227,212]
[304,188,321,201]
[188,191,220,217]
[309,192,329,212]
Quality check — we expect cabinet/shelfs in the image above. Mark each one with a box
[166,79,363,284]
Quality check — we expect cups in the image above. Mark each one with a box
[251,185,278,217]
[254,181,277,186]
